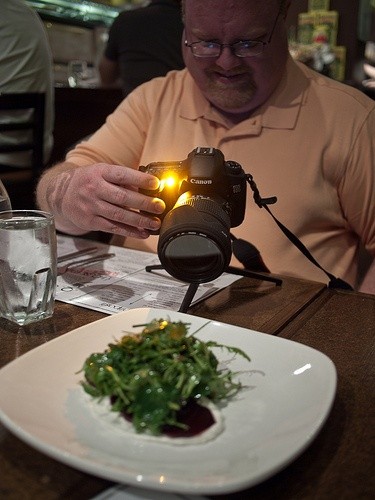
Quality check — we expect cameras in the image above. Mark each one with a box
[138,145,247,283]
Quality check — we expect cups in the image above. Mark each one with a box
[0,210,57,327]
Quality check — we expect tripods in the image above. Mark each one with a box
[143,264,283,315]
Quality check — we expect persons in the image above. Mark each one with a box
[0,0,54,210]
[99,0,186,103]
[36,0,375,293]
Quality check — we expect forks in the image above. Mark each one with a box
[58,253,115,276]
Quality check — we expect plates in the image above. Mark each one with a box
[0,308,337,495]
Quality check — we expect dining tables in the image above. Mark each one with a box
[0,240,375,500]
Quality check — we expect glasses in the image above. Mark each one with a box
[182,6,283,59]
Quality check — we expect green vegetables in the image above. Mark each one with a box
[74,313,252,436]
